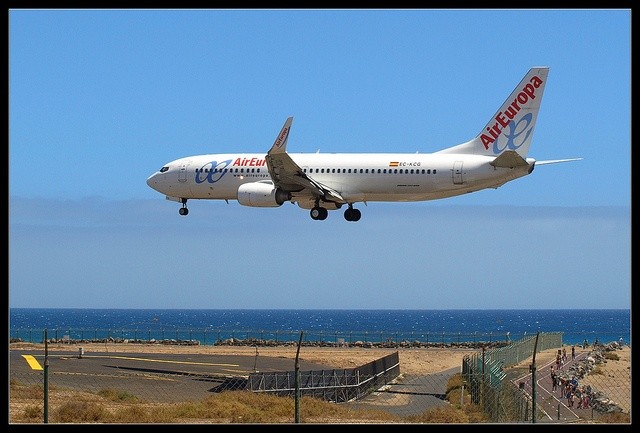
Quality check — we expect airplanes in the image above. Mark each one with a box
[146,65,585,222]
